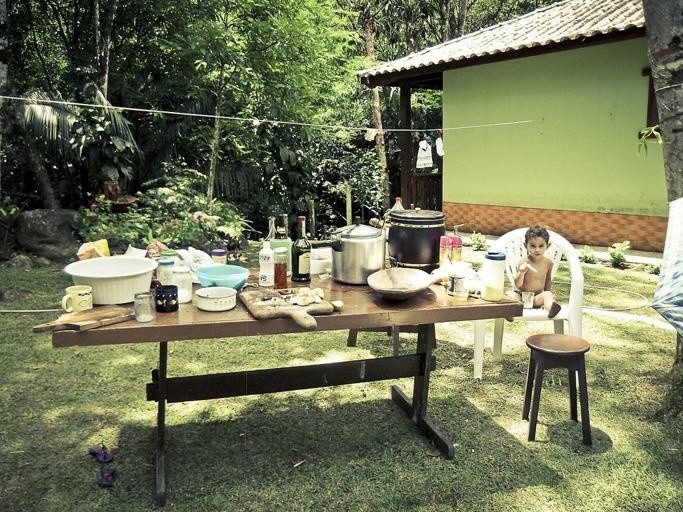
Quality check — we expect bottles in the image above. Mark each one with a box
[259,242,273,288]
[154,251,179,286]
[273,247,289,289]
[265,213,291,277]
[290,215,311,283]
[211,249,226,265]
[170,267,192,303]
[479,251,507,301]
[391,196,404,210]
[437,231,461,266]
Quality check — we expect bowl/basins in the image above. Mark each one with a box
[194,287,237,312]
[194,264,252,288]
[366,266,433,302]
[62,255,158,305]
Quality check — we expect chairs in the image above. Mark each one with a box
[473,227,584,390]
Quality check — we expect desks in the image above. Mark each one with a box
[52,271,523,505]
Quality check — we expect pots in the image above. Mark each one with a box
[385,207,445,270]
[311,216,386,287]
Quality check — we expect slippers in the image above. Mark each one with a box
[96,466,118,487]
[88,445,113,463]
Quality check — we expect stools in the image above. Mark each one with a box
[524,334,592,446]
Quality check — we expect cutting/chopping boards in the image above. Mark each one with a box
[239,287,334,332]
[36,303,135,333]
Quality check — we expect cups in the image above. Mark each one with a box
[522,292,534,309]
[61,285,93,312]
[454,277,469,301]
[134,292,155,322]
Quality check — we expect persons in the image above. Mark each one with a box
[502,225,562,322]
